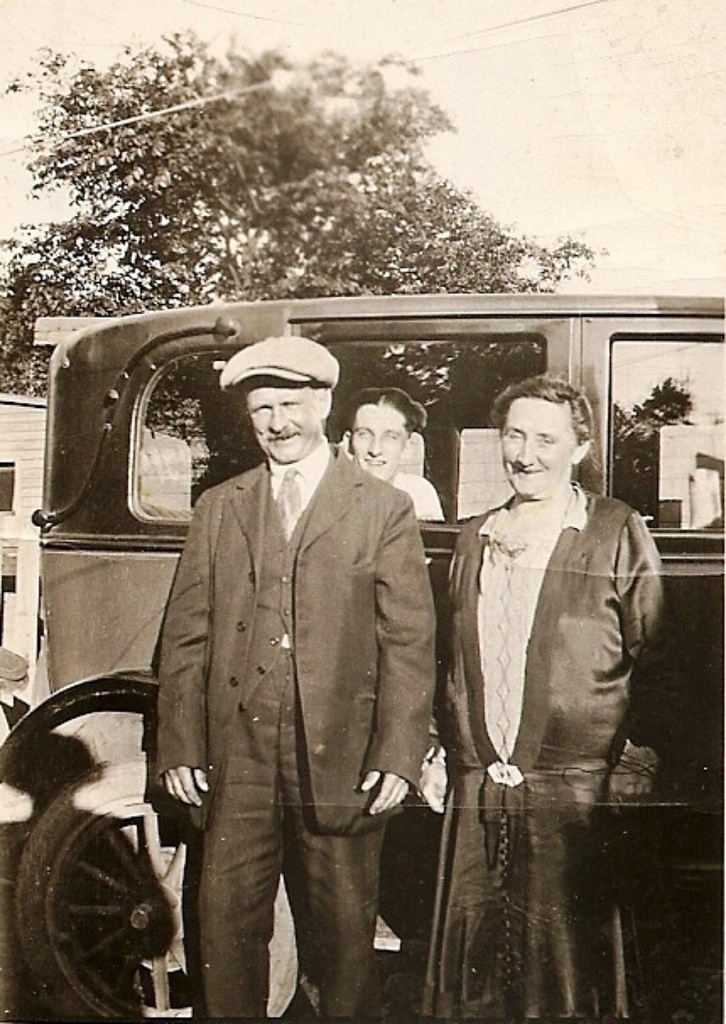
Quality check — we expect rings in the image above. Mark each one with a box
[610,795,624,805]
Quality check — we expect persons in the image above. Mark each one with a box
[339,386,447,523]
[158,336,433,1024]
[422,375,674,1024]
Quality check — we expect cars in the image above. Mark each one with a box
[0,292,723,1024]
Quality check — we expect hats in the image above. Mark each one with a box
[219,336,340,395]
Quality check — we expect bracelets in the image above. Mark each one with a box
[618,752,659,781]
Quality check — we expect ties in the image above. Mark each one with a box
[276,468,302,542]
